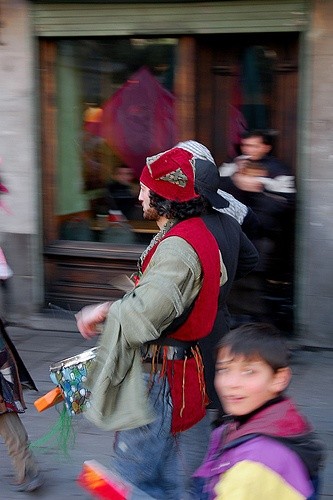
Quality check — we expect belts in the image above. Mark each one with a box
[141,342,200,360]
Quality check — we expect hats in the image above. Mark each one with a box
[146,140,216,169]
[141,148,198,202]
[194,157,229,208]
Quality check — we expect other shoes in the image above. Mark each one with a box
[9,474,44,492]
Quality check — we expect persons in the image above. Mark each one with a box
[180,323,325,500]
[173,139,260,475]
[219,129,297,327]
[98,161,145,217]
[75,148,228,500]
[0,319,44,492]
